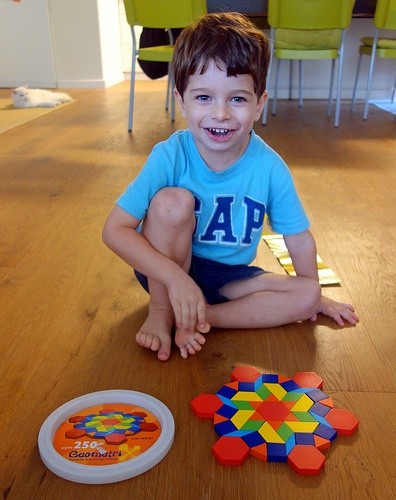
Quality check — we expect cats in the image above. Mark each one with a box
[11,84,73,108]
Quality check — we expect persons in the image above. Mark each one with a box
[102,11,360,361]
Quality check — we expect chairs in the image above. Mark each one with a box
[261,0,356,128]
[350,0,396,120]
[124,0,207,132]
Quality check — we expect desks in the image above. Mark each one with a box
[246,13,396,99]
